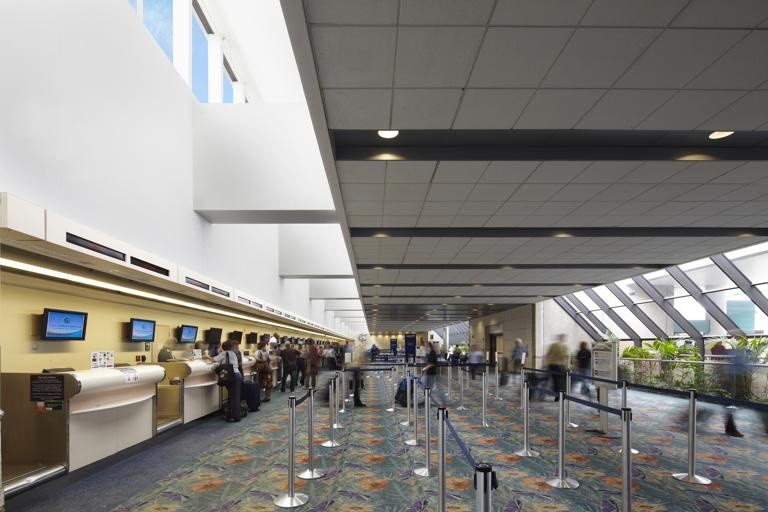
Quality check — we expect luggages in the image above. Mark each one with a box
[242,373,261,412]
[394,371,425,407]
[501,372,510,385]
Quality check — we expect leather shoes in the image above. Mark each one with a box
[357,404,366,407]
[727,429,745,437]
[262,399,270,402]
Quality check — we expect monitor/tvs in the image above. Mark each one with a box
[129,317,157,342]
[177,325,199,342]
[207,327,338,345]
[42,307,87,341]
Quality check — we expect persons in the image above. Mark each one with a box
[468,345,485,380]
[421,342,437,385]
[510,338,528,383]
[543,333,571,402]
[448,343,461,360]
[370,345,380,361]
[203,337,353,423]
[575,341,591,395]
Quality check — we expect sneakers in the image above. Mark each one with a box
[309,387,313,389]
[303,386,308,390]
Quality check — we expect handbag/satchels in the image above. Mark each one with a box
[222,400,248,419]
[316,358,323,367]
[215,364,234,387]
